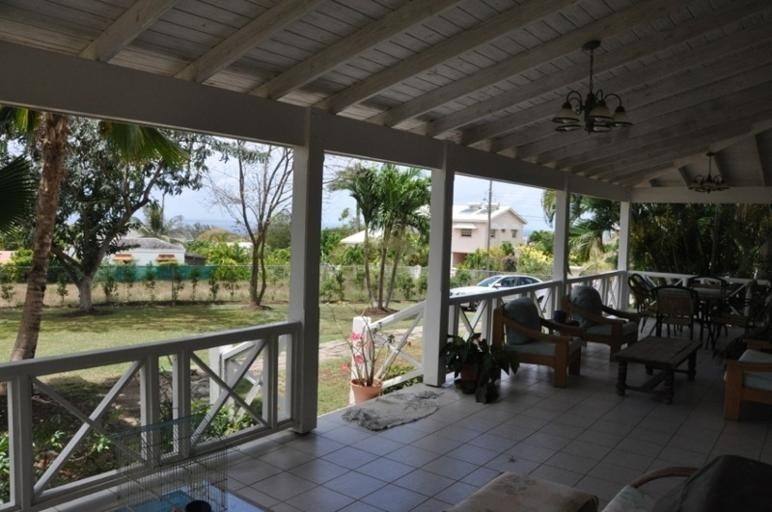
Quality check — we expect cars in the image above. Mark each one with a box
[450,273,550,312]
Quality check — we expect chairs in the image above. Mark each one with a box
[491,284,642,389]
[625,272,772,351]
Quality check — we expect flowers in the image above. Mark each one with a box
[340,312,393,388]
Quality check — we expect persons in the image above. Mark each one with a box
[502,251,518,272]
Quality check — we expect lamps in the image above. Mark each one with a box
[685,149,731,197]
[549,37,635,134]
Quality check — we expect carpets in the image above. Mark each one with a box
[340,389,440,434]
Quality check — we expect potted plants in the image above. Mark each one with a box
[438,331,519,405]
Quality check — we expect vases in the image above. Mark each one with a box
[350,376,384,404]
